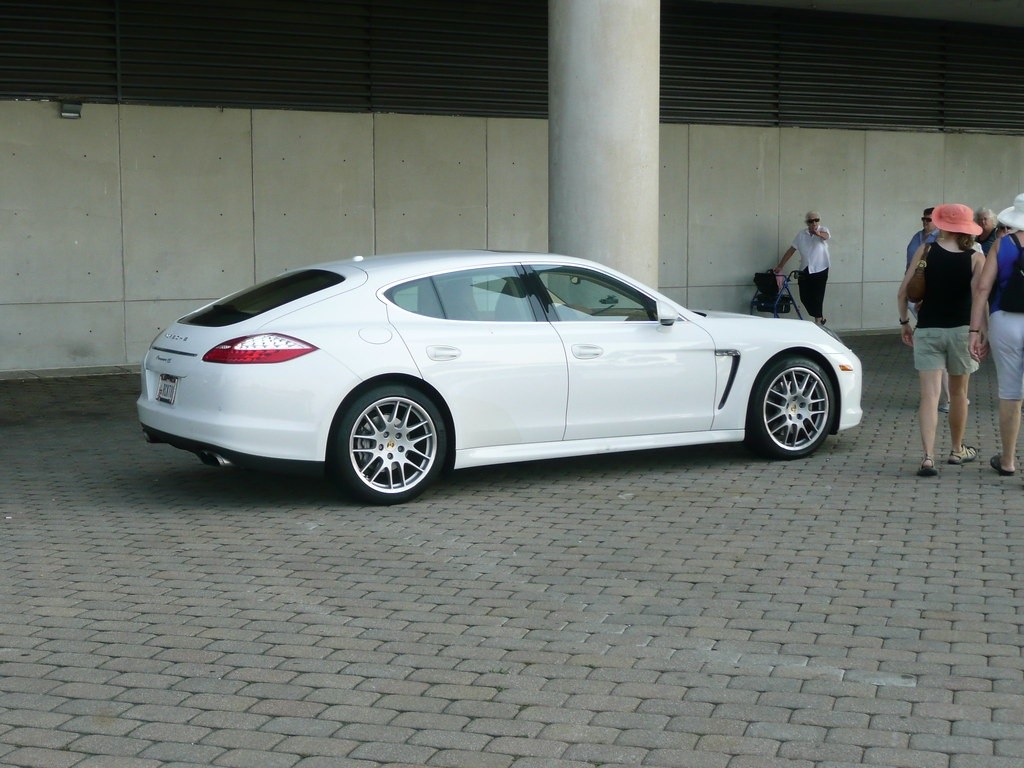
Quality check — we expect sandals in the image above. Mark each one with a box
[917,456,938,476]
[948,444,977,464]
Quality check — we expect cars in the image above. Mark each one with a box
[137,249,863,506]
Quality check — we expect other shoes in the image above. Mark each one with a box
[821,319,826,325]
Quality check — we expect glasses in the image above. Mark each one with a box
[922,217,932,222]
[808,219,819,223]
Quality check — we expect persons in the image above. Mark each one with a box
[774,212,832,325]
[898,193,1024,475]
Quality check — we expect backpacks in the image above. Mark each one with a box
[995,234,1024,313]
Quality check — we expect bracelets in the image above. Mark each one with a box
[968,330,979,333]
[900,317,910,324]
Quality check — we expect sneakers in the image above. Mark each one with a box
[939,401,950,413]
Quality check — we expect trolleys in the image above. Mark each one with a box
[750,268,806,321]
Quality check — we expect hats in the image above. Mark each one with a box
[997,194,1024,230]
[932,204,983,235]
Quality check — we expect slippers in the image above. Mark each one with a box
[990,456,1013,475]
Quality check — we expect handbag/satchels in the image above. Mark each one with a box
[753,269,779,296]
[759,297,790,313]
[908,243,930,303]
